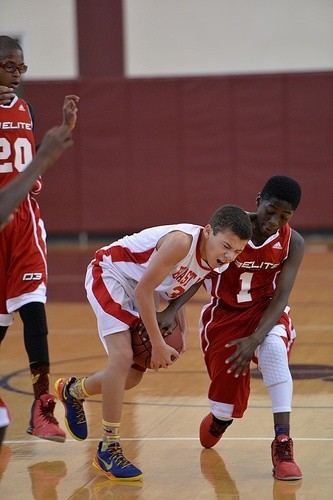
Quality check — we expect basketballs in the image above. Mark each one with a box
[131,319,183,369]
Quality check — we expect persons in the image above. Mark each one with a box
[55,204,253,481]
[0,35,80,442]
[158,176,305,480]
[0,86,75,446]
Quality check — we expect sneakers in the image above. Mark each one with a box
[271,434,302,480]
[274,479,303,493]
[54,377,88,440]
[93,441,144,481]
[27,394,66,443]
[199,413,234,448]
[28,461,65,485]
[200,448,229,483]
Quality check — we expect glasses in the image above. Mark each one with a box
[0,61,28,74]
